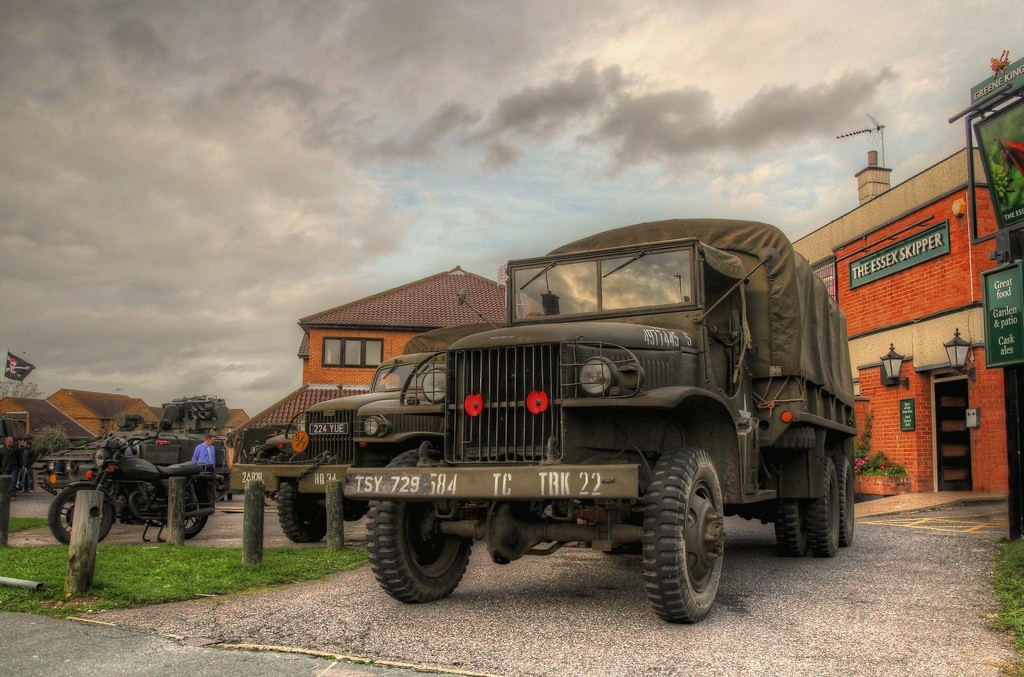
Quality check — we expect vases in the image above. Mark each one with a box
[854,476,911,495]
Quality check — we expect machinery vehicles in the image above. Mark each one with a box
[29,394,231,503]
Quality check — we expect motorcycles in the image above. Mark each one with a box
[45,402,223,547]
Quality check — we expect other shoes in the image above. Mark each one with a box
[18,488,28,493]
[11,496,16,501]
[28,489,35,493]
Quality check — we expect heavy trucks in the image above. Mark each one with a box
[226,320,508,546]
[341,216,859,626]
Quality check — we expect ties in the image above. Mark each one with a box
[206,445,213,464]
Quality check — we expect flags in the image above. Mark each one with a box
[4,352,35,383]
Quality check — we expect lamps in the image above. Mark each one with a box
[943,328,977,380]
[881,344,909,389]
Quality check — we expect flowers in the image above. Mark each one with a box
[853,450,909,477]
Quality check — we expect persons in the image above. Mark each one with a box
[192,433,216,474]
[0,435,38,501]
